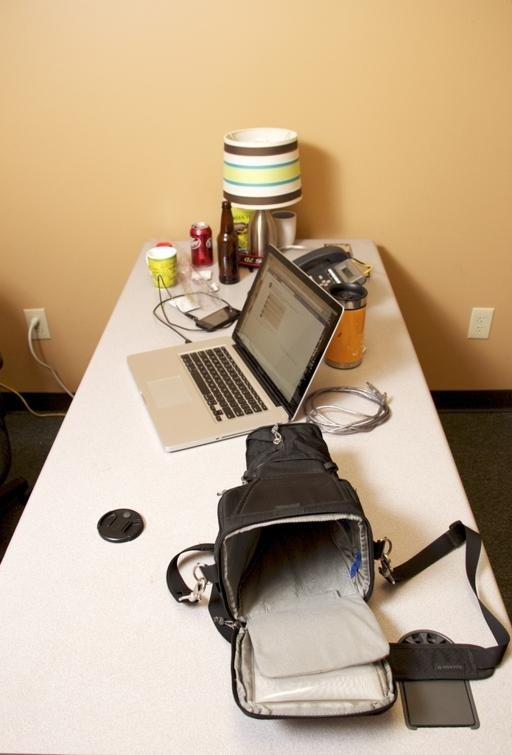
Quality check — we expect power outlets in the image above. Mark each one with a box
[466,308,494,339]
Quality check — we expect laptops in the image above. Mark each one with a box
[127,241,344,454]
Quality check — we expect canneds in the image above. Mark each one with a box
[191,222,213,266]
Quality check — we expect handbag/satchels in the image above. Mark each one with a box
[161,421,511,720]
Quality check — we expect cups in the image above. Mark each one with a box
[145,246,178,289]
[323,282,369,370]
[272,210,297,251]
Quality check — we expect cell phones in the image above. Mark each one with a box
[196,306,241,331]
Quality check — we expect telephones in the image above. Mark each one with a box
[293,245,368,293]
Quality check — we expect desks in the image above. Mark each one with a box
[0,238,510,754]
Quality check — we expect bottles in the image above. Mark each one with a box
[216,199,240,285]
